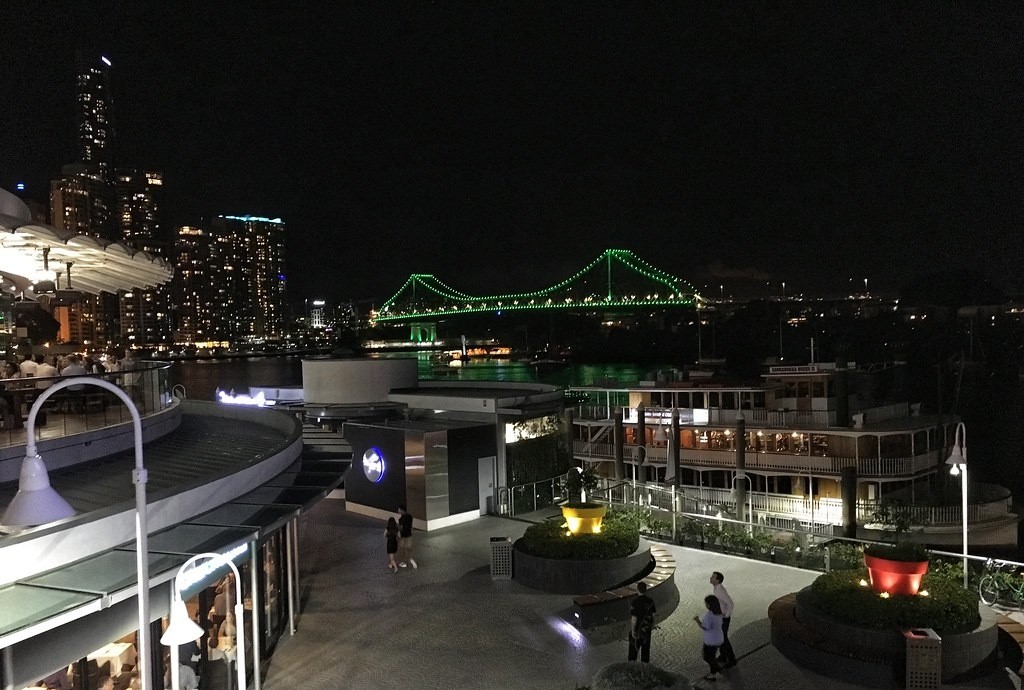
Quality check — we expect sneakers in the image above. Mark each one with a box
[410,559,417,569]
[399,562,407,567]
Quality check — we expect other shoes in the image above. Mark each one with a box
[716,655,725,662]
[723,662,737,669]
[387,564,393,568]
[726,670,730,679]
[704,676,716,682]
[394,568,398,574]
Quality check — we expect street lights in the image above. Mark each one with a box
[728,473,755,553]
[944,421,970,593]
[159,552,250,690]
[2,377,153,690]
[654,407,683,546]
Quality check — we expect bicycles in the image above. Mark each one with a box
[978,559,1024,614]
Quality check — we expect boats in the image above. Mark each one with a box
[430,336,549,370]
[572,336,1020,563]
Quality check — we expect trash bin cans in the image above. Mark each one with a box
[896,628,941,690]
[489,536,513,580]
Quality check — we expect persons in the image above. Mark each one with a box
[398,504,418,570]
[384,517,402,572]
[20,531,286,689]
[1,328,173,429]
[694,595,725,681]
[628,582,657,663]
[709,571,738,668]
